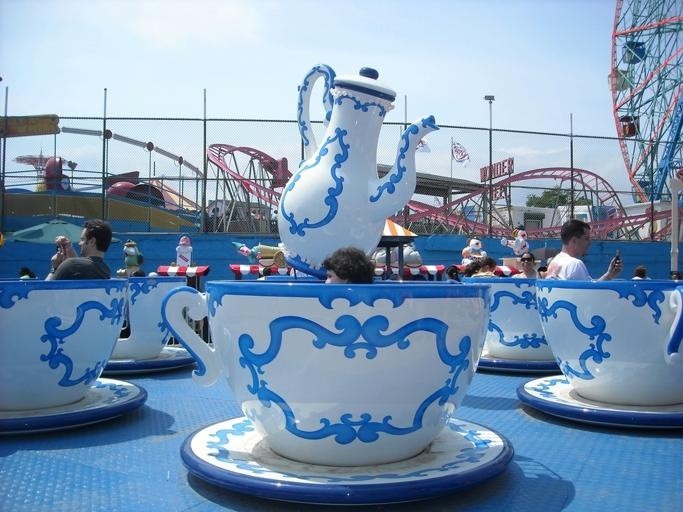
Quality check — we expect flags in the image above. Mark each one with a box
[414,139,431,155]
[450,138,470,169]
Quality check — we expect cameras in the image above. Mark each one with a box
[56,244,67,254]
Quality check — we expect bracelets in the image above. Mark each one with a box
[49,268,55,273]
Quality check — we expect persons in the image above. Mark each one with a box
[545,217,624,281]
[631,264,653,280]
[441,250,556,284]
[321,246,375,284]
[41,218,111,281]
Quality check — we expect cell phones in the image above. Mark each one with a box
[614,249,620,267]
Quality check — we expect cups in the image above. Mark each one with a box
[160,278,493,468]
[461,275,564,362]
[0,276,129,414]
[101,273,190,361]
[531,278,683,408]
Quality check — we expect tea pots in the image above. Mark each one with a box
[276,62,441,282]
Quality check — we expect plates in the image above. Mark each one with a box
[472,357,565,377]
[179,414,515,508]
[0,375,150,436]
[514,373,683,431]
[93,343,209,377]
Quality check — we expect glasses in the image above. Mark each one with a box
[521,257,533,262]
